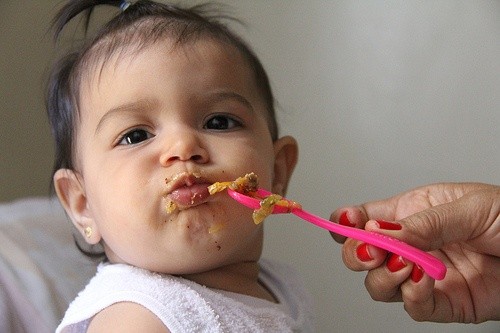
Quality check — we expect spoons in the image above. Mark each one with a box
[227,186,448,282]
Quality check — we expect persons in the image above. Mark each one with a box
[41,0,318,333]
[329,181,500,322]
